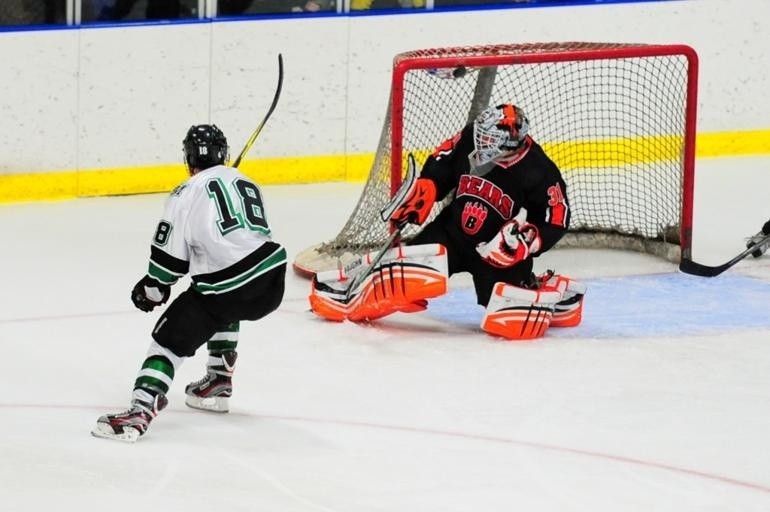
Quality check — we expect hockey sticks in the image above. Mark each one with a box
[679,238,768,276]
[316,223,410,304]
[233,53,284,168]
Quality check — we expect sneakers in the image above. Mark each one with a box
[746,230,770,258]
[96,386,168,436]
[185,349,238,398]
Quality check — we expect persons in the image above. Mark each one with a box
[392,102,571,307]
[93,123,287,435]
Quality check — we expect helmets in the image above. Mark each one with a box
[181,123,228,170]
[468,103,529,176]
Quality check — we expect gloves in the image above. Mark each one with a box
[390,178,436,232]
[130,274,171,313]
[482,219,540,270]
